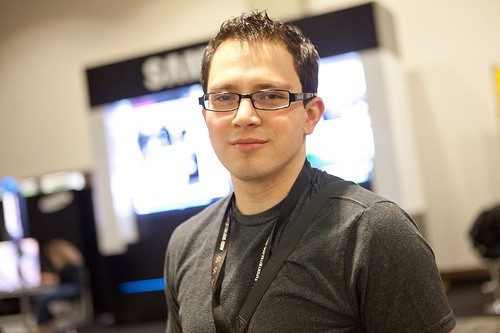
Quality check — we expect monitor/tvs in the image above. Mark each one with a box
[80,2,410,323]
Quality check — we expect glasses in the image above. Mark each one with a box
[198,88,318,112]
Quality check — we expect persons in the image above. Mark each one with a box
[162,14,460,333]
[29,238,83,330]
